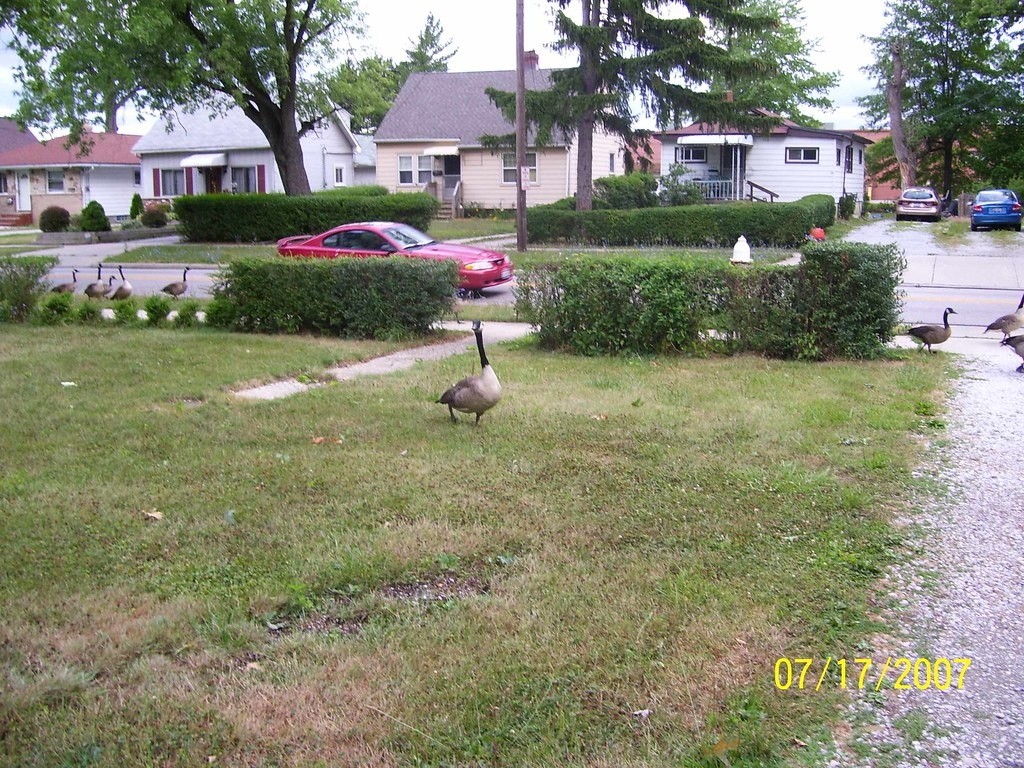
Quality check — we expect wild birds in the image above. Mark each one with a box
[905,308,958,354]
[983,293,1024,375]
[435,319,502,424]
[160,267,191,300]
[51,269,80,293]
[84,264,133,300]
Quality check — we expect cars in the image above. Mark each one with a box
[895,188,941,222]
[276,222,516,292]
[967,189,1023,231]
